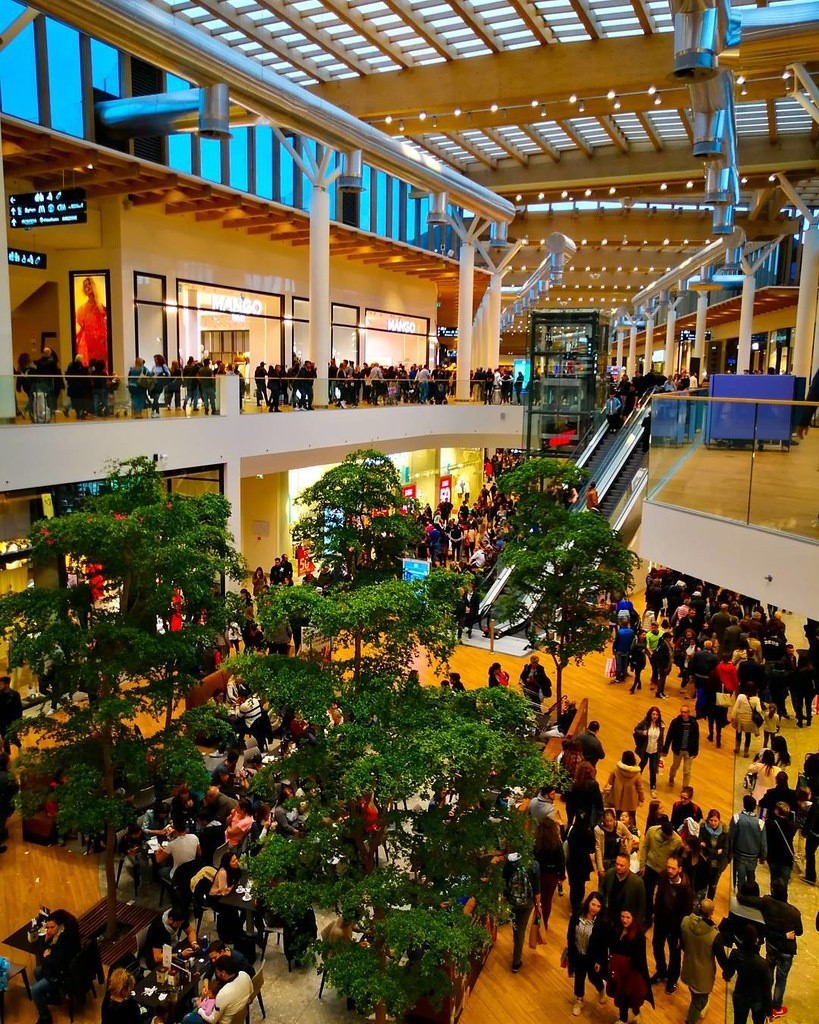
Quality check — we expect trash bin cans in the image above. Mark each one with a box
[32,391,52,423]
[492,388,501,405]
[706,371,793,442]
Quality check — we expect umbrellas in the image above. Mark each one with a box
[732,858,738,894]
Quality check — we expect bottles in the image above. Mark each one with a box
[202,936,208,954]
[558,882,563,897]
[177,949,183,959]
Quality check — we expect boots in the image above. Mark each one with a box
[734,741,740,752]
[743,747,749,758]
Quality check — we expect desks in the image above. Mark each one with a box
[130,935,217,1015]
[0,961,32,1024]
[1,917,52,967]
[221,885,259,934]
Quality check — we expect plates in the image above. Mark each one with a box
[242,895,252,901]
[236,888,245,893]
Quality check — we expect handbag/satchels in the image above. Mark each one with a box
[752,709,763,728]
[562,839,569,859]
[530,906,547,949]
[600,783,615,808]
[793,851,803,874]
[605,656,616,677]
[560,948,569,968]
[715,692,732,707]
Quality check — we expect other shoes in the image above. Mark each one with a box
[716,741,721,748]
[669,776,674,786]
[651,789,656,798]
[656,692,669,699]
[512,959,523,971]
[666,978,677,994]
[799,875,816,884]
[649,971,667,983]
[634,1013,642,1024]
[707,735,713,742]
[699,999,709,1019]
[599,989,606,1003]
[614,1019,629,1024]
[573,1000,584,1015]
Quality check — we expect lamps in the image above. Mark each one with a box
[620,207,631,219]
[645,206,657,220]
[384,71,792,131]
[516,210,529,224]
[572,208,579,220]
[548,208,553,219]
[699,207,709,220]
[597,207,604,218]
[673,207,683,218]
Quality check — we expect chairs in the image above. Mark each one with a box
[54,793,410,1024]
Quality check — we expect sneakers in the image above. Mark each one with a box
[770,1005,788,1017]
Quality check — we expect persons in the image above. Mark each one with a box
[76,278,107,377]
[13,347,776,432]
[338,447,598,576]
[796,368,819,440]
[0,554,819,1024]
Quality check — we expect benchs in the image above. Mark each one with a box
[185,669,232,748]
[20,728,157,847]
[542,697,589,764]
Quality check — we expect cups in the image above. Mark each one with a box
[238,885,243,892]
[245,893,252,899]
